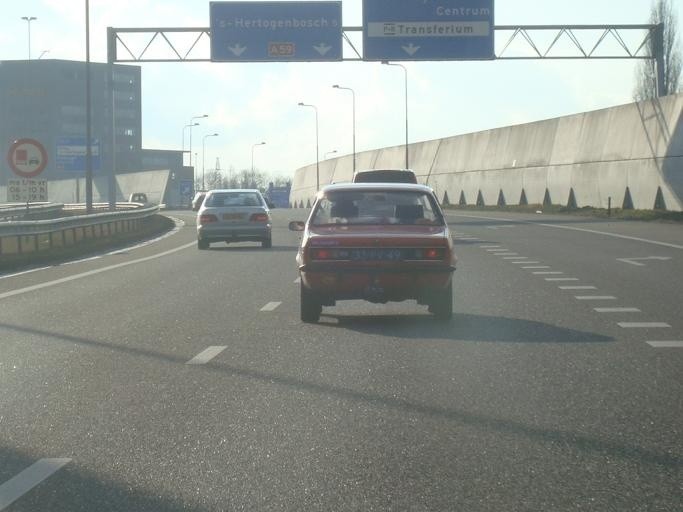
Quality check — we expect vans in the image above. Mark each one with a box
[353,168,424,222]
[127,192,146,204]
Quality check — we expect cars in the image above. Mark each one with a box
[190,190,206,210]
[286,181,456,322]
[194,188,272,250]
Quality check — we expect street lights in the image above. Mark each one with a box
[251,142,266,183]
[189,115,208,166]
[324,150,336,160]
[297,103,320,191]
[21,16,37,61]
[202,134,218,190]
[332,85,355,179]
[182,123,200,153]
[380,60,408,169]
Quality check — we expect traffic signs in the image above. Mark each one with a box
[209,0,342,62]
[361,0,496,61]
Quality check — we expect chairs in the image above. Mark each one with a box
[209,197,257,207]
[331,204,424,223]
